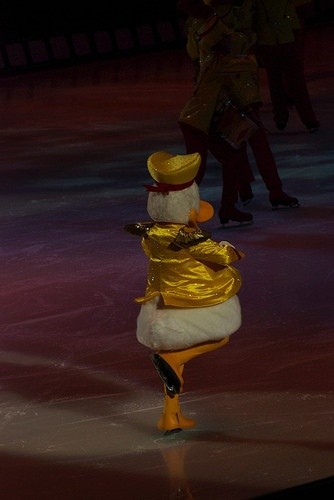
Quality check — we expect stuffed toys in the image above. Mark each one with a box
[126,150,245,435]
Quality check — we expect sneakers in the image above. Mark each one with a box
[306,120,320,132]
[218,207,253,228]
[269,189,299,209]
[241,194,253,205]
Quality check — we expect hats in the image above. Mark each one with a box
[194,16,234,48]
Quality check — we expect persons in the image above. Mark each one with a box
[177,0,299,225]
[251,0,321,132]
